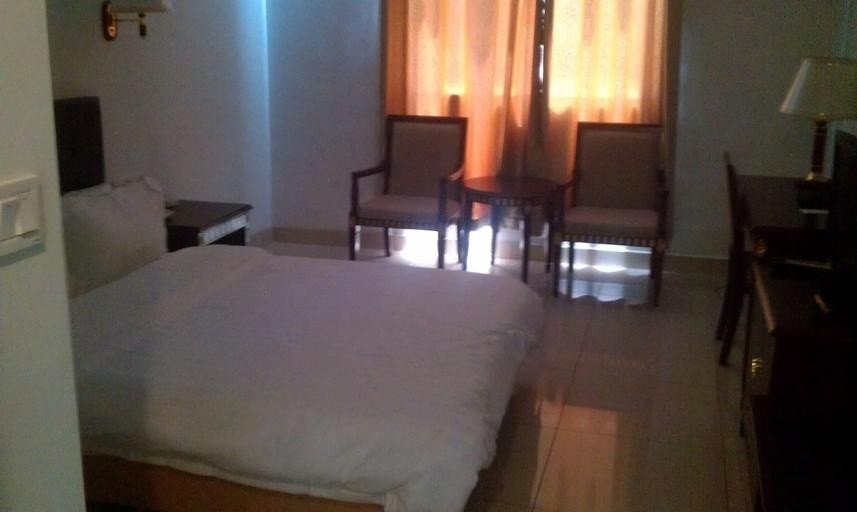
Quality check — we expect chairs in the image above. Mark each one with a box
[349,113,468,270]
[717,152,740,369]
[552,122,668,307]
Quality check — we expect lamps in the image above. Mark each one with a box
[779,58,855,210]
[99,0,169,40]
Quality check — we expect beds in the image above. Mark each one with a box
[67,243,542,510]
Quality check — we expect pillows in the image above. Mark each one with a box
[61,176,167,302]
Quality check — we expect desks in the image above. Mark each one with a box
[461,175,556,285]
[740,176,855,510]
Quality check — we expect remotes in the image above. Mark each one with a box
[770,250,834,272]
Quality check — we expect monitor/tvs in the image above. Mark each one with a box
[832,130,857,293]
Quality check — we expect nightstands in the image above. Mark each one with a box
[165,199,253,251]
